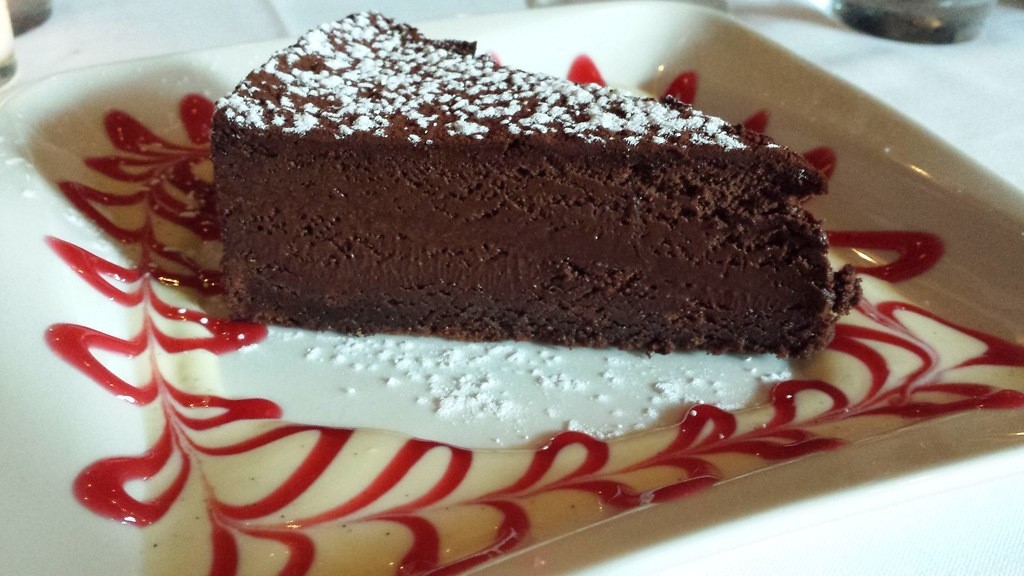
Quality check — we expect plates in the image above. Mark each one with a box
[0,0,1024,576]
